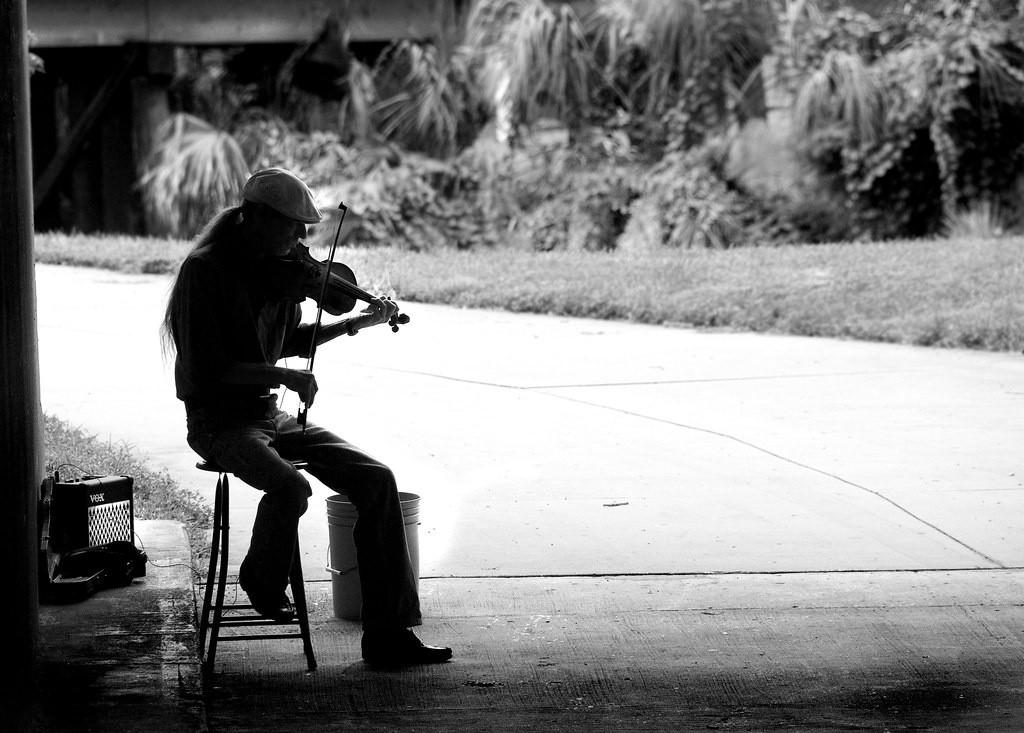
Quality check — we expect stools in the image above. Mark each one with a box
[196,460,318,702]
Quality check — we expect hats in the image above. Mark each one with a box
[242,166,322,225]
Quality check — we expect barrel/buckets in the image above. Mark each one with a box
[324,491,421,622]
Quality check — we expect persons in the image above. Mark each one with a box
[160,166,453,665]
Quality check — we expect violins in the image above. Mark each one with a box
[279,242,410,332]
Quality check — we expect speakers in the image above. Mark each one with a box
[49,474,134,554]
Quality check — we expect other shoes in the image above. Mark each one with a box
[361,627,452,666]
[238,567,295,622]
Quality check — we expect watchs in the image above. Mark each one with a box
[346,317,358,336]
